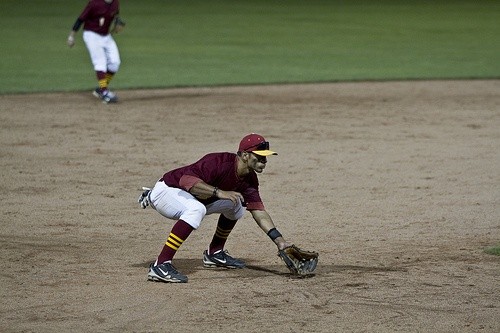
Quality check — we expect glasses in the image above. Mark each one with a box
[244,142,269,151]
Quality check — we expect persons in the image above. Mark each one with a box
[136,131,319,283]
[66,0,128,103]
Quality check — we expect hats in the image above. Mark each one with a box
[238,134,278,156]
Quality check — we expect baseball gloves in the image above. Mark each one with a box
[278,244,319,277]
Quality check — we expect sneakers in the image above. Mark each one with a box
[147,260,188,283]
[101,92,118,105]
[202,250,246,269]
[92,87,102,99]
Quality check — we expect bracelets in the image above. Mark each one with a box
[211,185,219,200]
